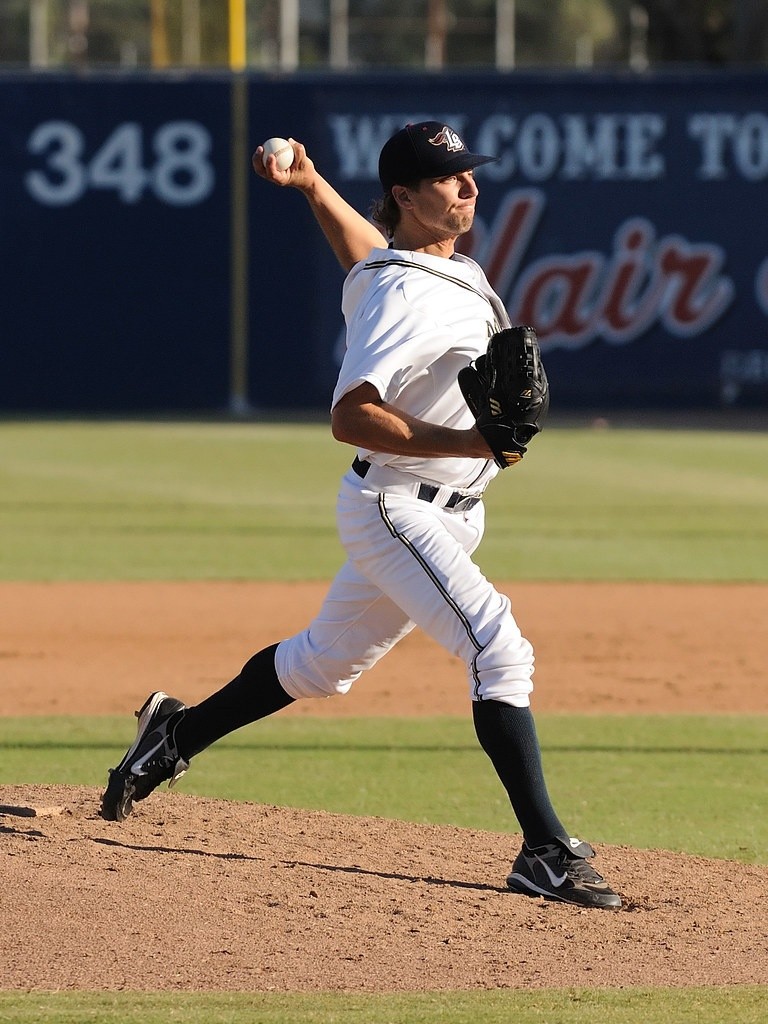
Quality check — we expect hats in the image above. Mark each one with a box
[379,121,498,192]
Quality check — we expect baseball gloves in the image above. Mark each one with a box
[456,325,551,470]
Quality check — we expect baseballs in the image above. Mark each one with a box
[262,137,295,171]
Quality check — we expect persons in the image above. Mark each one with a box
[98,120,622,908]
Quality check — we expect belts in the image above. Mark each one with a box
[351,454,480,510]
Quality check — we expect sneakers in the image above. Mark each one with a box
[100,690,189,821]
[506,836,622,908]
